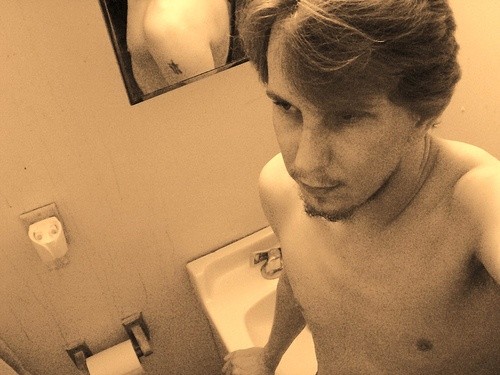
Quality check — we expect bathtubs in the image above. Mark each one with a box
[184,226,318,374]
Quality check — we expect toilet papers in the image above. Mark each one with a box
[85,338,146,375]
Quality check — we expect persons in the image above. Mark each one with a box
[222,0,500,375]
[126,1,230,95]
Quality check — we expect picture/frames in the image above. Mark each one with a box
[98,0,252,107]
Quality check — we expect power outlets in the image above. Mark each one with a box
[18,202,72,249]
